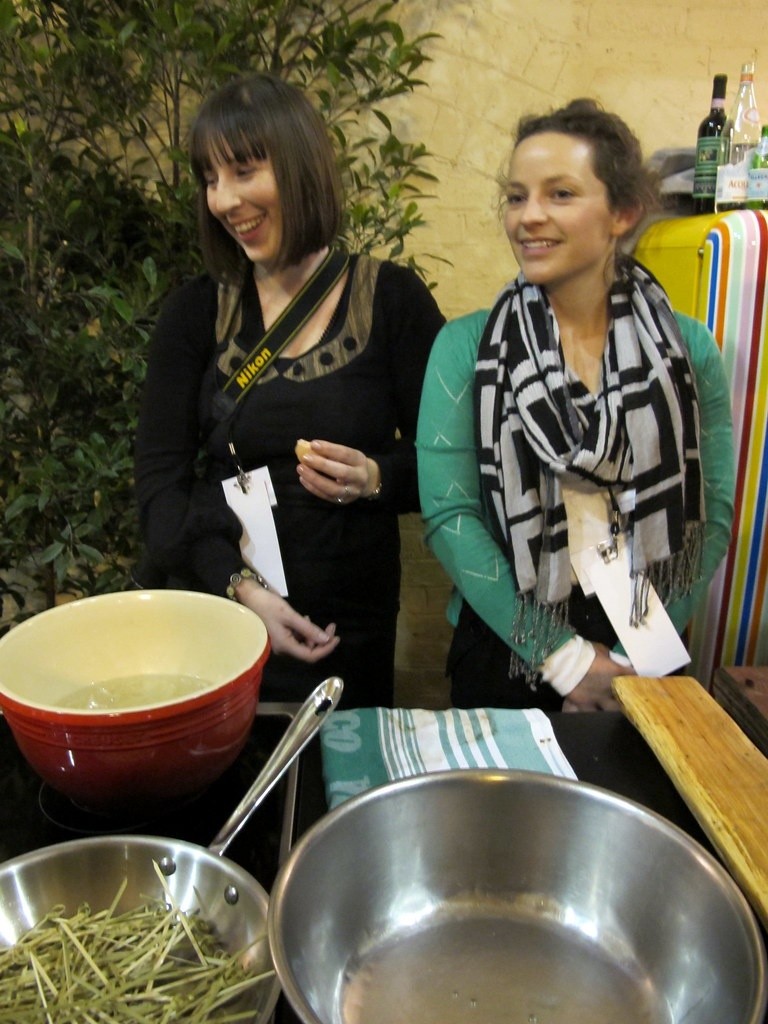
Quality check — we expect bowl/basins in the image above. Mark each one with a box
[267,768,768,1024]
[0,589,271,808]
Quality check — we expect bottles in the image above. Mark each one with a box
[717,62,768,212]
[692,77,727,214]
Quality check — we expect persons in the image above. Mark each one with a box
[132,82,447,712]
[418,96,735,715]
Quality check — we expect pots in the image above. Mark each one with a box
[0,677,346,1024]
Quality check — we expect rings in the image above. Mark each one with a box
[337,486,351,505]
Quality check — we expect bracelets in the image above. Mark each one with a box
[369,483,383,501]
[227,568,269,603]
